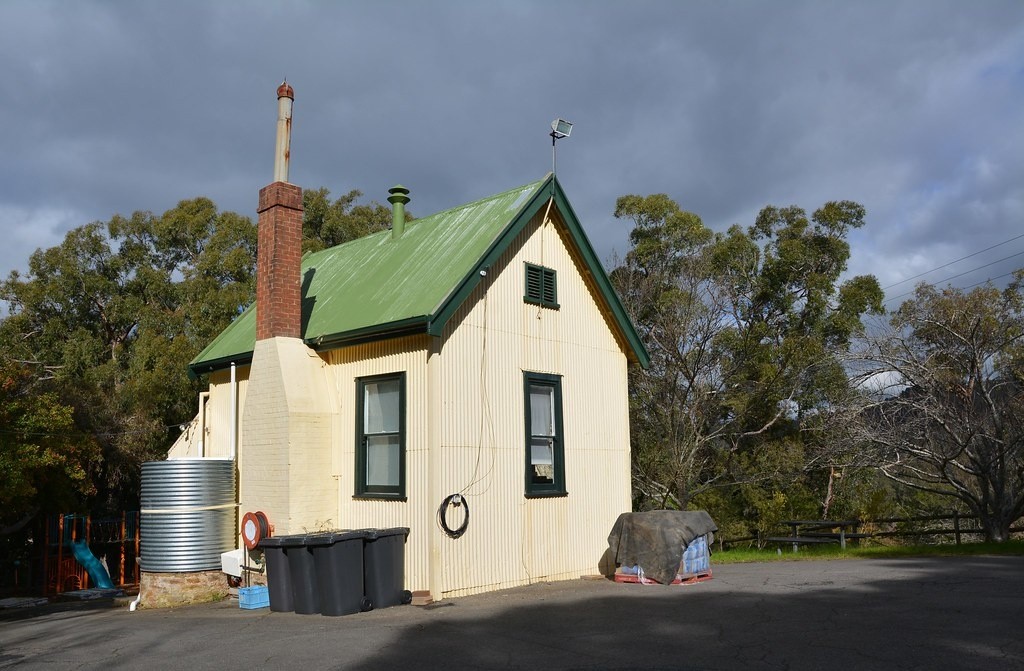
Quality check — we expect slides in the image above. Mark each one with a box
[72,540,114,590]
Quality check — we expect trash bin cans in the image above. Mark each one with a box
[262,527,413,617]
